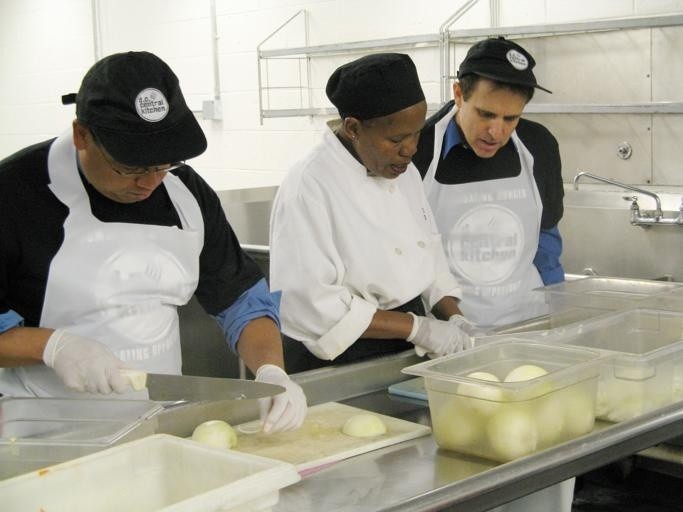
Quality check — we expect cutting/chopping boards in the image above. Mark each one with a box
[183,401,431,472]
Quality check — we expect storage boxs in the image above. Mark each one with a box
[1,432,302,512]
[531,276,683,423]
[0,396,165,482]
[402,338,609,465]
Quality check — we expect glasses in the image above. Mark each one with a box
[88,128,186,175]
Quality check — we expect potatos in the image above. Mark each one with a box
[192,418,237,449]
[342,413,385,437]
[436,364,595,460]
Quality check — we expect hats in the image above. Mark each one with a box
[325,52,425,121]
[458,35,552,93]
[62,51,207,167]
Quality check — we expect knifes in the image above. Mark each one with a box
[117,369,286,401]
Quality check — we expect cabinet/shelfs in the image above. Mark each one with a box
[445,1,683,126]
[255,2,471,123]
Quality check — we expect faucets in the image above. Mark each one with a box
[573,172,662,214]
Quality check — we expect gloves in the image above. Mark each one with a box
[405,311,476,359]
[255,365,307,432]
[43,328,128,395]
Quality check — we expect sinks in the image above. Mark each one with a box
[651,275,683,283]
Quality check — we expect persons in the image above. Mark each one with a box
[0,51,308,435]
[268,50,478,372]
[408,36,566,331]
